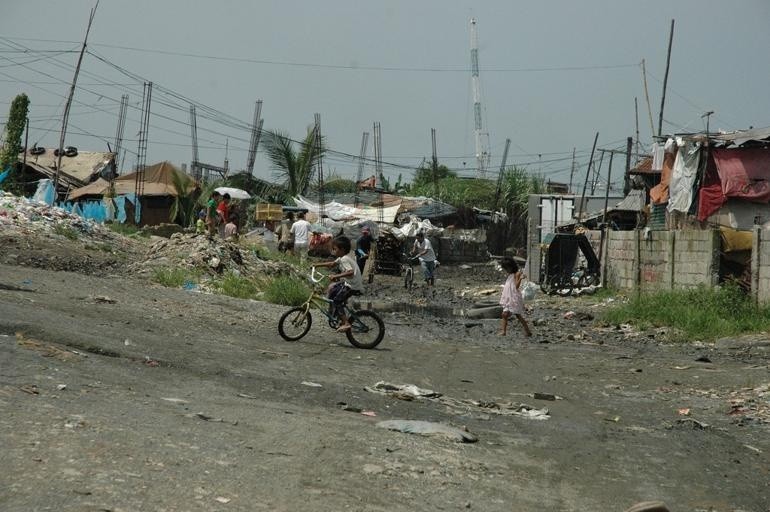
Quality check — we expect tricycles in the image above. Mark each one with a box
[539,264,598,296]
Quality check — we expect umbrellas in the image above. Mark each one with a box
[215,186,251,203]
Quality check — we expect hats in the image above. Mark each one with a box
[361,226,369,233]
[199,211,207,217]
[297,211,304,218]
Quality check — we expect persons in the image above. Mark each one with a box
[356,225,371,276]
[309,237,364,332]
[290,213,311,280]
[196,190,243,266]
[499,255,533,337]
[274,212,296,256]
[409,233,436,286]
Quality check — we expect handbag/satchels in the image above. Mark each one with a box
[522,280,535,300]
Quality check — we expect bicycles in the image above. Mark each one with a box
[401,252,420,292]
[274,262,387,352]
